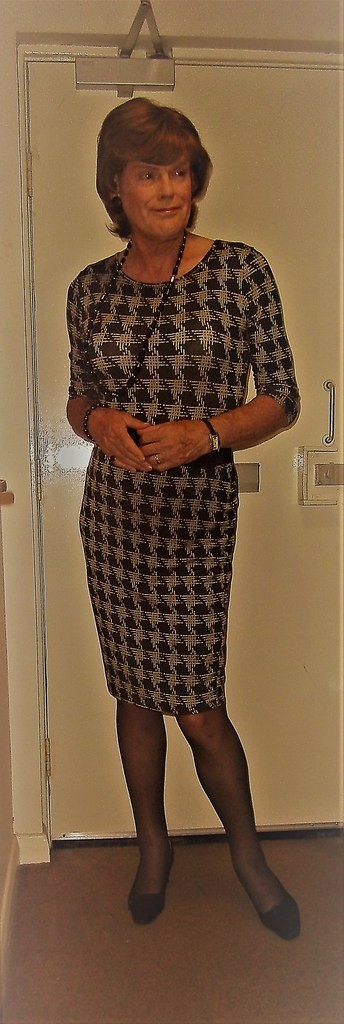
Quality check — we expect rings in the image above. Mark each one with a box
[108,455,115,463]
[155,454,160,464]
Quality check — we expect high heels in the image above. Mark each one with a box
[241,864,298,939]
[128,839,174,924]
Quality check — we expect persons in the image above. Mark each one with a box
[65,98,302,939]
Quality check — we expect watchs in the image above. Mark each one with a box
[202,418,220,452]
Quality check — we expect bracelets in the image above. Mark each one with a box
[83,403,108,443]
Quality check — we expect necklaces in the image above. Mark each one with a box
[84,227,186,398]
